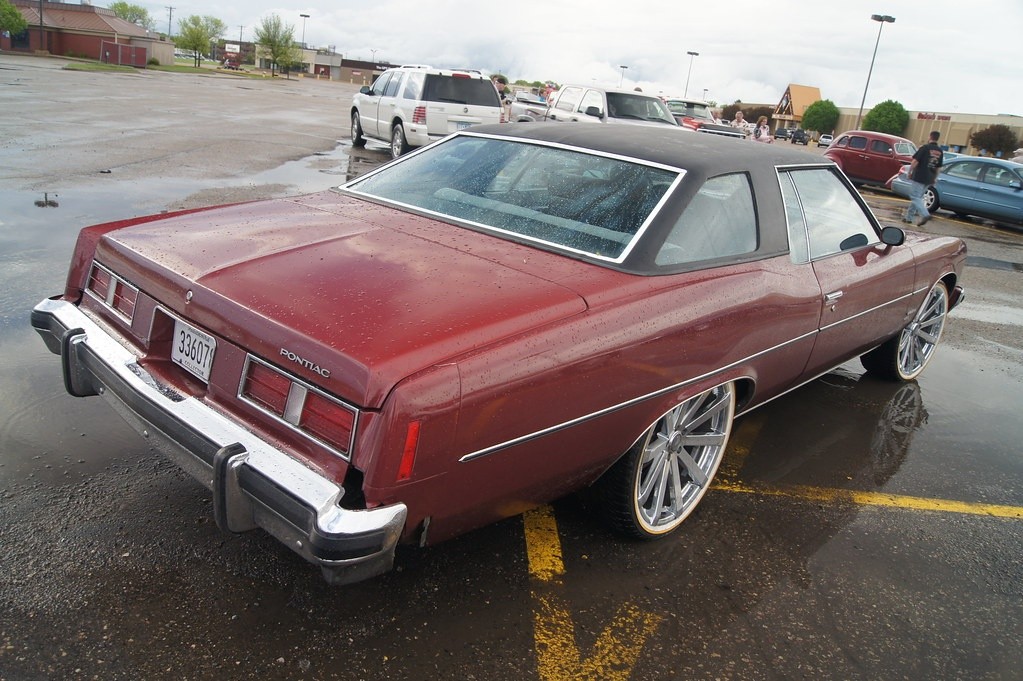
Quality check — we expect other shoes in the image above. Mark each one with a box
[917,215,933,226]
[902,219,912,223]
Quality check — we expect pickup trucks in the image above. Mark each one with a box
[224,58,239,69]
[507,85,677,127]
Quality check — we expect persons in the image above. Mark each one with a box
[713,110,722,124]
[538,89,547,101]
[752,116,773,143]
[902,131,943,226]
[493,75,506,101]
[1011,148,1023,177]
[730,111,750,135]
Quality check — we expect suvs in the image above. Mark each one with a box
[774,128,787,140]
[790,129,809,145]
[349,65,505,160]
[818,134,833,148]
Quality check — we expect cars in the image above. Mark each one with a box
[941,150,999,178]
[787,130,794,138]
[892,156,1023,225]
[32,121,970,588]
[664,99,745,139]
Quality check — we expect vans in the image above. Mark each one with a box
[821,129,916,188]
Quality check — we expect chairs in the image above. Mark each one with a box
[615,103,636,116]
[1000,172,1012,186]
[641,103,658,117]
[546,171,619,245]
[987,170,997,185]
[636,182,733,261]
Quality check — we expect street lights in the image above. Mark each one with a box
[619,66,628,89]
[299,14,310,74]
[704,88,708,99]
[684,52,699,99]
[854,14,896,130]
